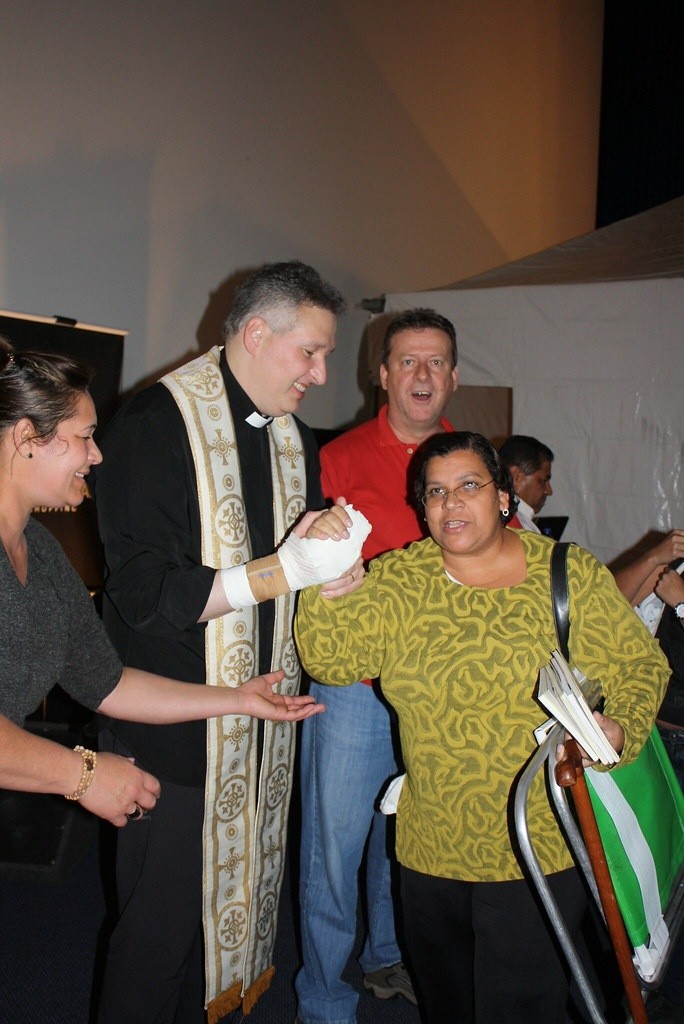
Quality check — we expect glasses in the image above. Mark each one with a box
[421,480,495,508]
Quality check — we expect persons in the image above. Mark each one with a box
[1,334,328,1024]
[616,529,684,795]
[90,261,373,1024]
[296,306,521,1024]
[292,430,673,1023]
[502,435,554,534]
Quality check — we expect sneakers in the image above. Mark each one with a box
[363,963,418,1006]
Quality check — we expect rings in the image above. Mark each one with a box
[350,573,355,582]
[126,805,140,819]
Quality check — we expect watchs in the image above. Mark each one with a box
[674,602,684,619]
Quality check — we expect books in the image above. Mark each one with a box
[538,649,622,766]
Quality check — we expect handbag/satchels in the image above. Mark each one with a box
[549,542,684,980]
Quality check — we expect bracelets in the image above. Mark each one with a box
[65,744,97,800]
